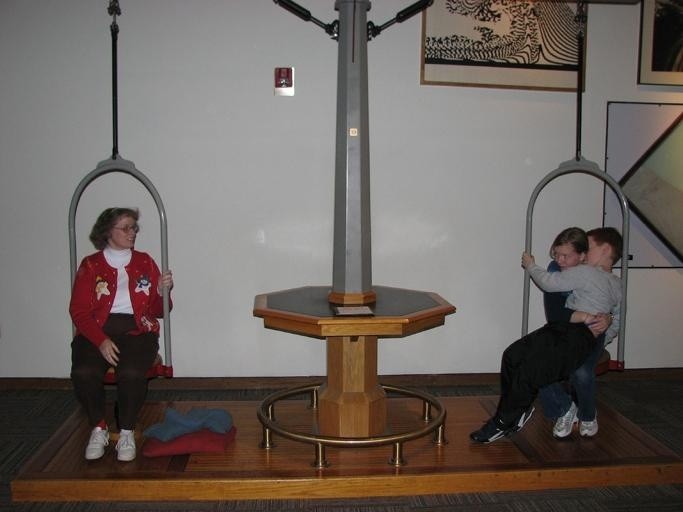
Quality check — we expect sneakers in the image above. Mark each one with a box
[553,401,579,438]
[580,410,599,436]
[469,405,535,444]
[85,424,110,459]
[115,429,136,461]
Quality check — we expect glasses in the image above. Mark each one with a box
[115,225,139,232]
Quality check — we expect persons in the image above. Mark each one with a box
[469,227,623,445]
[69,207,174,462]
[537,227,612,441]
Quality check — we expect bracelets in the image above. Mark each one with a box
[561,313,584,323]
[609,313,615,321]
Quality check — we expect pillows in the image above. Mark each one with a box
[141,419,236,456]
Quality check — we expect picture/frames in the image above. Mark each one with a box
[617,104,683,263]
[637,0,683,85]
[420,0,585,93]
